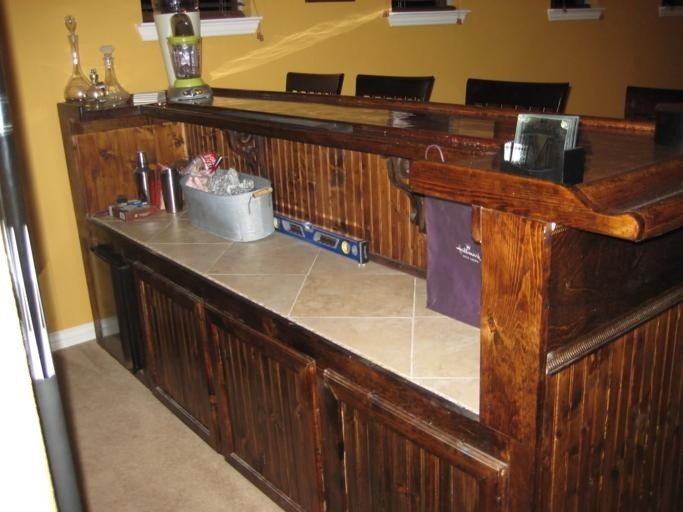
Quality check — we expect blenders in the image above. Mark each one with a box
[149,0,213,105]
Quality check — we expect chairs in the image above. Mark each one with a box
[466,78,569,113]
[356,75,435,102]
[286,73,344,95]
[625,86,683,145]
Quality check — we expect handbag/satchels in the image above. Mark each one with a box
[423,142,480,331]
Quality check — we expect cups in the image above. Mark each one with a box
[159,168,183,215]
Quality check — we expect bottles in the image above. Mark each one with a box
[63,15,130,110]
[133,151,151,203]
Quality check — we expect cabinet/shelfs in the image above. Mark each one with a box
[127,261,208,453]
[204,302,327,512]
[326,365,507,511]
